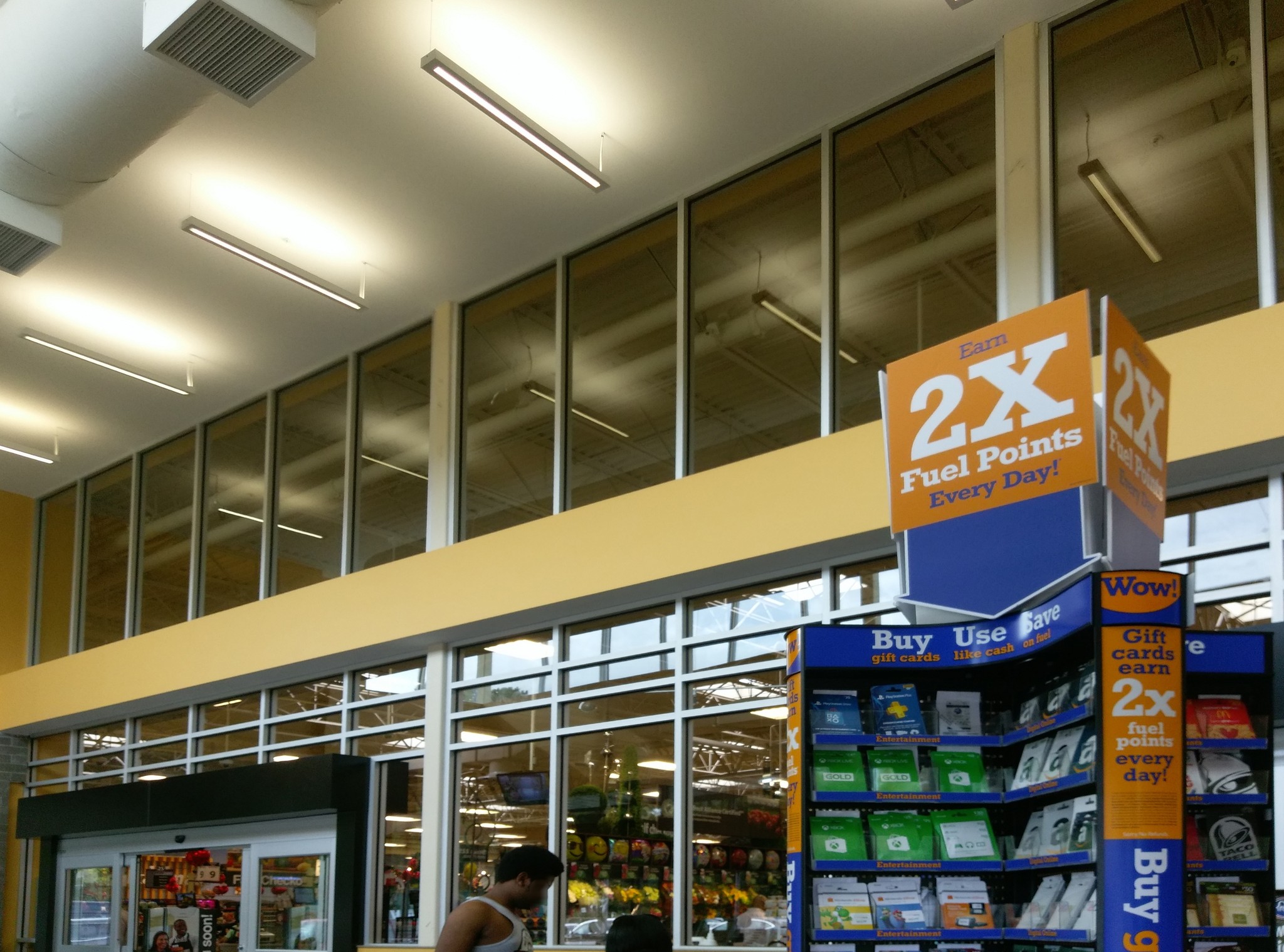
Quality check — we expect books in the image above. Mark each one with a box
[810,684,1259,952]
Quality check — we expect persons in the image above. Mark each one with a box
[435,845,564,952]
[218,920,240,944]
[148,919,199,952]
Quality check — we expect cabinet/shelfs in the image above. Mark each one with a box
[785,571,1279,952]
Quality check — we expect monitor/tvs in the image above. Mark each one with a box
[497,771,550,806]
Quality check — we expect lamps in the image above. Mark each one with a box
[1078,112,1165,268]
[752,253,865,367]
[0,438,61,465]
[20,329,197,396]
[181,217,367,310]
[421,49,613,193]
[524,346,631,441]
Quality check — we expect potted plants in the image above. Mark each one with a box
[568,786,607,832]
[614,746,642,836]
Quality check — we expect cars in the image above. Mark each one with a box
[562,917,616,946]
[691,917,788,948]
